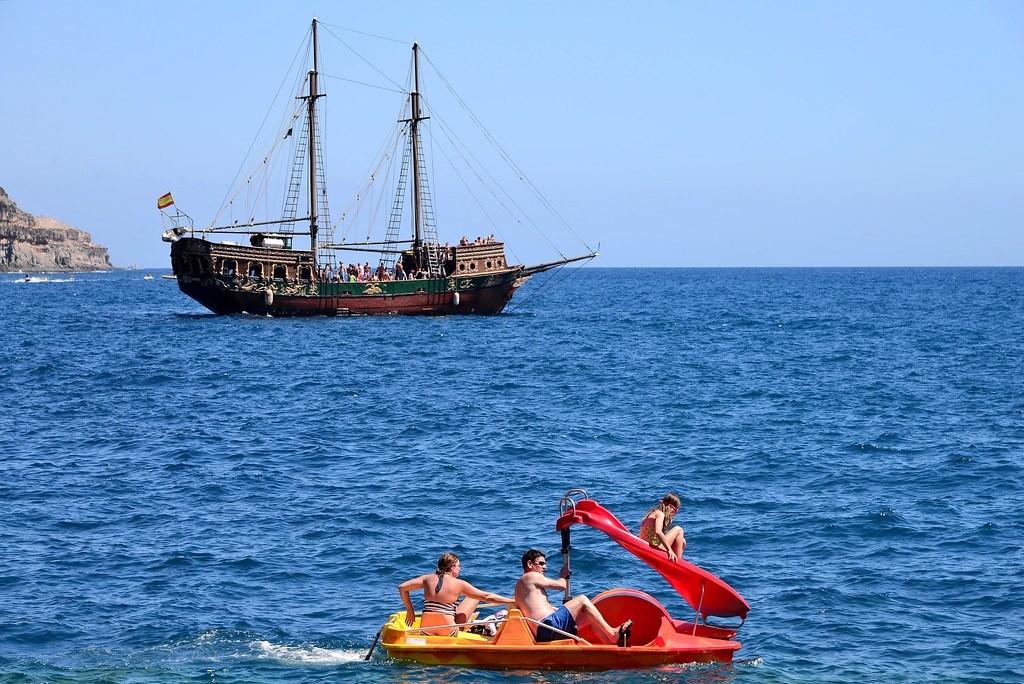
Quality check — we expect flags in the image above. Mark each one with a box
[157,192,175,209]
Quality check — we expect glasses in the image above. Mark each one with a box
[534,561,546,566]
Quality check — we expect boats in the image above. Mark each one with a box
[364,487,751,667]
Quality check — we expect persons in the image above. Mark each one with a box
[144,273,152,278]
[398,552,516,637]
[316,260,429,283]
[516,550,633,648]
[419,235,489,261]
[639,493,687,564]
[25,275,51,281]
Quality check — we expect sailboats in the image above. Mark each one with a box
[151,17,605,321]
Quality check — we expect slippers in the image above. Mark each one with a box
[615,620,634,648]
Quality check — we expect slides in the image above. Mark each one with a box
[556,501,751,621]
